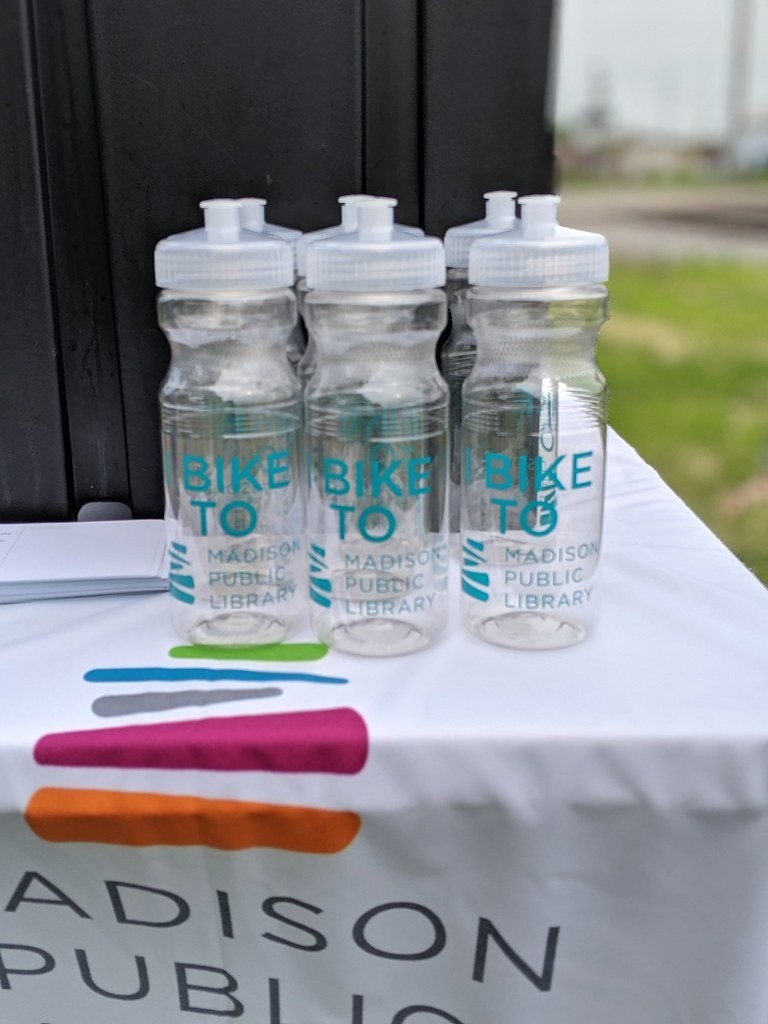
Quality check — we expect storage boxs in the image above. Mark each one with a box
[0,0,557,535]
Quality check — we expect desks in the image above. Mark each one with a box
[0,355,768,1024]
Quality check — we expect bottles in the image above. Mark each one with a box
[460,194,610,651]
[305,197,448,658]
[294,196,426,602]
[154,198,306,647]
[176,198,303,605]
[442,190,560,567]
